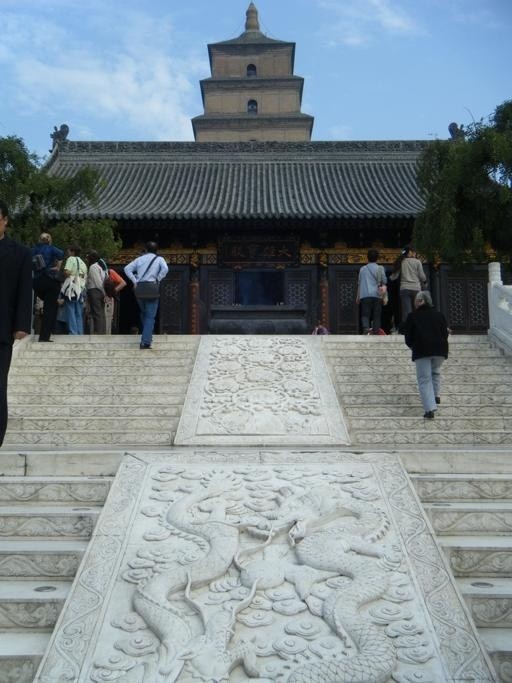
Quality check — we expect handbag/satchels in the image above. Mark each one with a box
[104,277,118,298]
[60,277,83,302]
[136,282,161,299]
[377,283,387,302]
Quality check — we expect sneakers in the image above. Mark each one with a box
[39,338,54,342]
[423,410,434,418]
[435,396,440,403]
[140,340,151,348]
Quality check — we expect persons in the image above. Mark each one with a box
[124,240,169,350]
[0,201,30,446]
[392,245,427,334]
[356,249,388,335]
[405,291,448,419]
[29,232,126,342]
